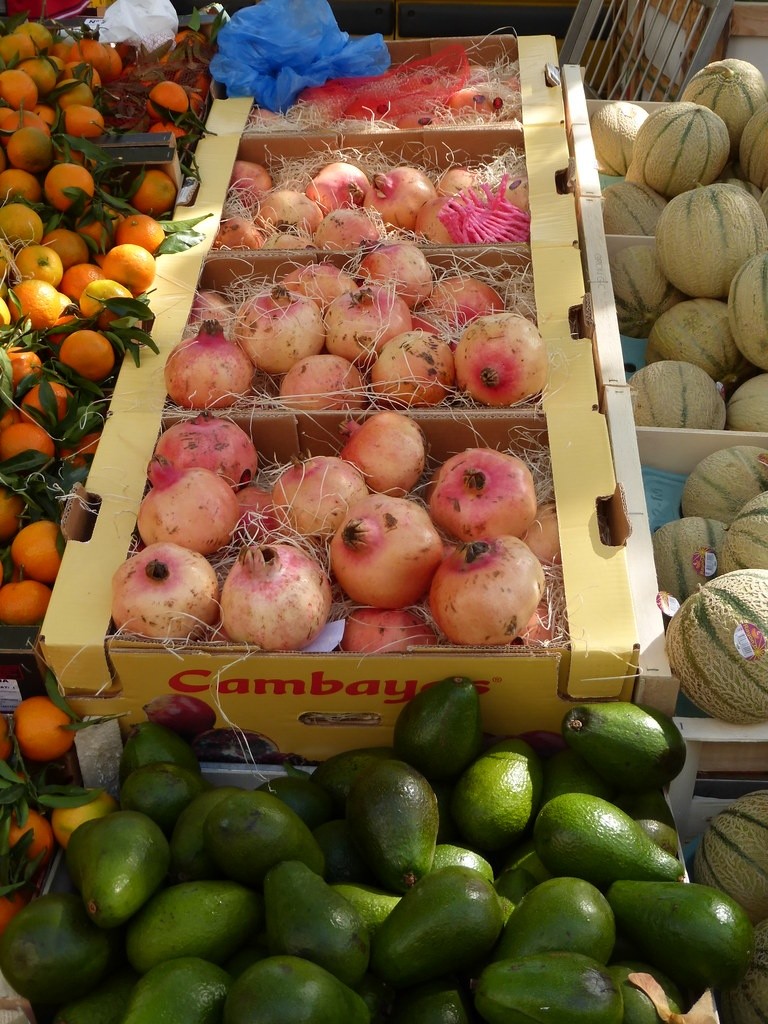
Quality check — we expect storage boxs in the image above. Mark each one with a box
[38,33,768,857]
